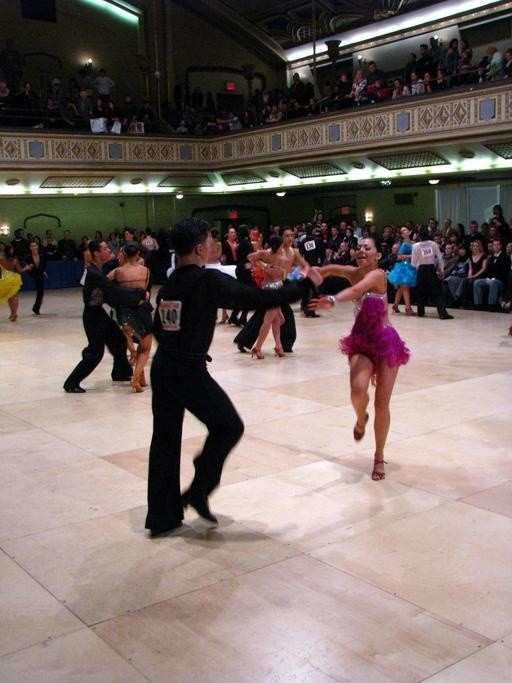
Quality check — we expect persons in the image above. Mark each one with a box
[1,36,512,131]
[307,233,411,482]
[141,215,323,536]
[0,206,511,393]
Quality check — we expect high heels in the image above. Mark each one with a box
[274,346,285,357]
[251,348,264,359]
[131,379,148,393]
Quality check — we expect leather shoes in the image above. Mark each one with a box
[72,388,84,393]
[238,344,246,353]
[183,490,218,524]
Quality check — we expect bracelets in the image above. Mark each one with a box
[324,291,337,310]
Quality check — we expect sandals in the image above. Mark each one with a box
[353,413,369,440]
[372,459,385,481]
